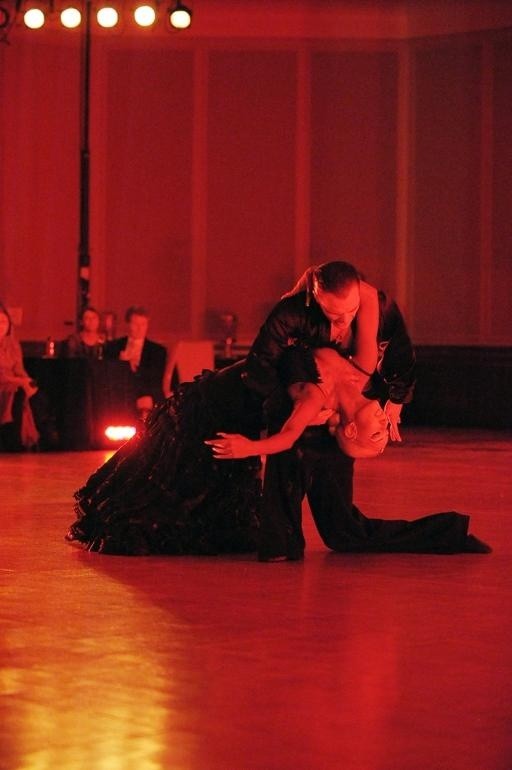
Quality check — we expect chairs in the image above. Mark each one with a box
[173,340,219,387]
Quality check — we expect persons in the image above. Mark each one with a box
[1,303,168,453]
[254,259,492,562]
[65,265,390,555]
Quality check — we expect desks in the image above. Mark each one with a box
[28,354,134,451]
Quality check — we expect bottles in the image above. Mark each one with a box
[45,336,58,356]
